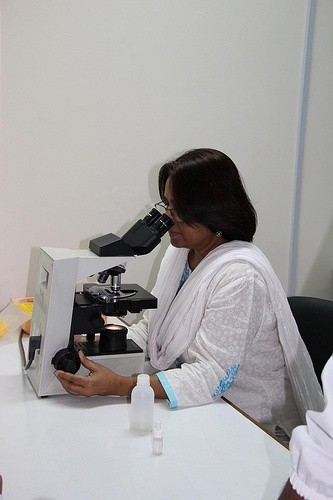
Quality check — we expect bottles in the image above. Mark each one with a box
[130,374,155,439]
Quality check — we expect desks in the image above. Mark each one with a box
[0,295,304,500]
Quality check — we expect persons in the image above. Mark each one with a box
[54,149,325,453]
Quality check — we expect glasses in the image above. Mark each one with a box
[154,200,184,223]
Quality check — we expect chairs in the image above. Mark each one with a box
[285,294,333,397]
